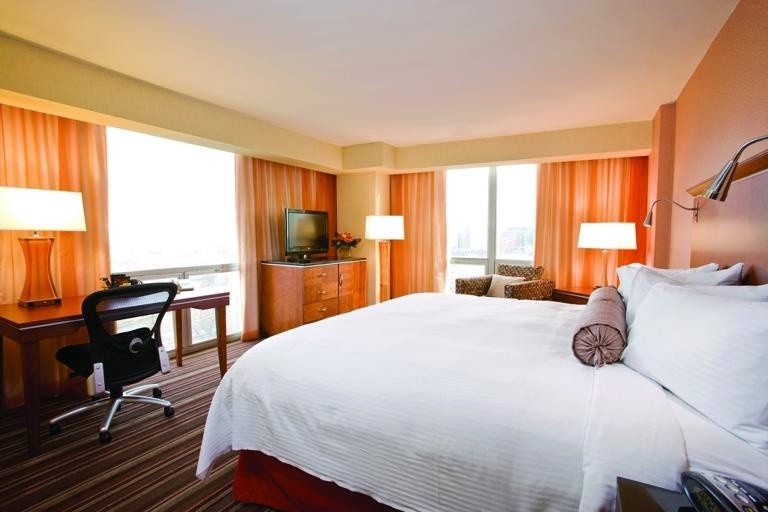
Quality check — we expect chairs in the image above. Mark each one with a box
[453,262,555,301]
[48,282,184,445]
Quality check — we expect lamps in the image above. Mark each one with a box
[575,222,637,286]
[0,186,86,308]
[703,134,766,202]
[642,199,698,228]
[364,215,404,305]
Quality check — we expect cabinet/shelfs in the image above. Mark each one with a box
[261,255,367,339]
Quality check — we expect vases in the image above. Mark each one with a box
[339,245,351,259]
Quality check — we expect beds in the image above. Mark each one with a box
[192,151,767,512]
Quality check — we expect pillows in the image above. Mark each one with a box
[486,273,526,298]
[570,257,767,455]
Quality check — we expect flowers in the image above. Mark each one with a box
[330,230,361,251]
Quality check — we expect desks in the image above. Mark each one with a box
[1,284,233,456]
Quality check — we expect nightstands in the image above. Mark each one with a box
[552,285,594,305]
[610,472,694,512]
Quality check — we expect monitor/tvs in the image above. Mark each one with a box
[284,207,329,263]
